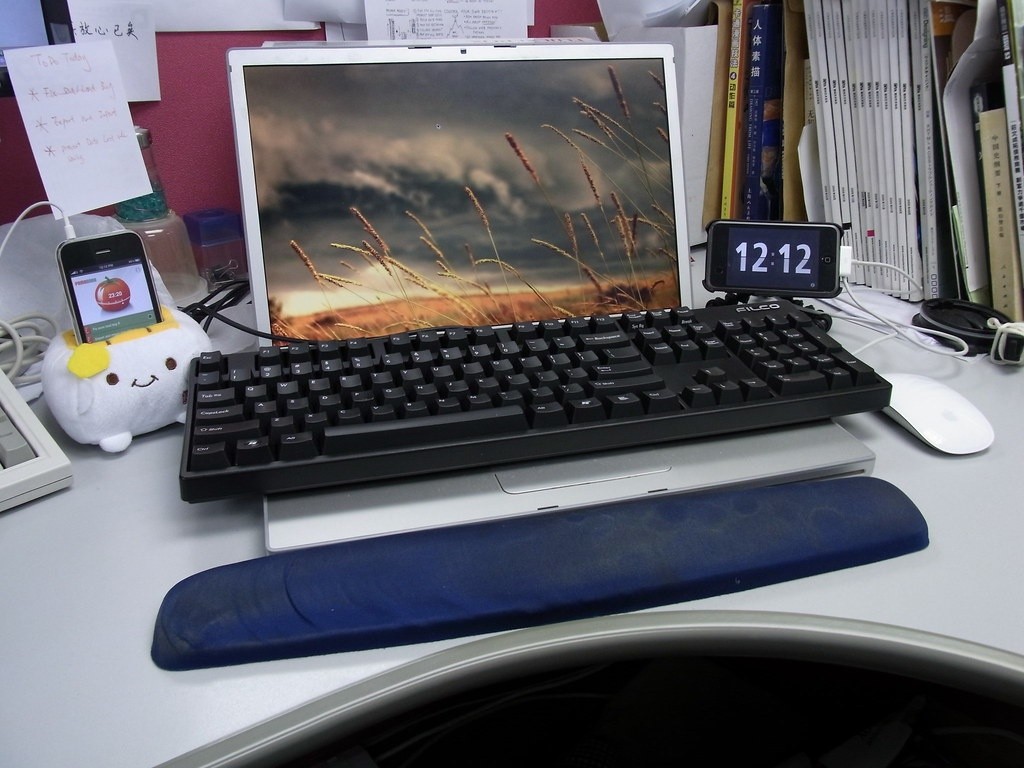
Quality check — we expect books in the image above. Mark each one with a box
[721,1,1022,324]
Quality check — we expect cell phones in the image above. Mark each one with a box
[56,228,165,345]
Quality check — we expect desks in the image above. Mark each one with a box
[0,215,1024,768]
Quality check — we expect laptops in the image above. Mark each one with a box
[228,42,877,554]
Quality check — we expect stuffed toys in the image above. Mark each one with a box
[40,303,214,453]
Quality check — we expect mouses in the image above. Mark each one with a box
[877,374,996,454]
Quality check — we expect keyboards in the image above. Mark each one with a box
[179,300,894,505]
[0,368,74,509]
